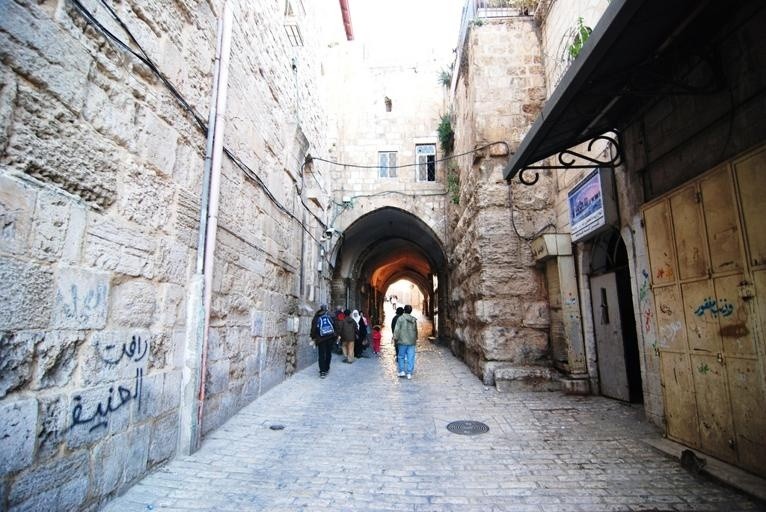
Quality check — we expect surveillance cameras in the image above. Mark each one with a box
[325,228,335,237]
[341,195,352,204]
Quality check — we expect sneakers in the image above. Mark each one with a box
[320,370,328,379]
[398,371,412,379]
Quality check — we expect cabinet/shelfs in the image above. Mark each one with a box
[642,197,678,290]
[723,356,766,479]
[681,280,722,353]
[691,354,738,464]
[751,266,766,360]
[662,351,697,445]
[712,274,755,355]
[732,148,766,267]
[696,163,748,275]
[653,285,687,349]
[667,185,708,282]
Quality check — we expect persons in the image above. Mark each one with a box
[391,307,405,363]
[332,305,380,364]
[310,305,336,378]
[392,305,418,380]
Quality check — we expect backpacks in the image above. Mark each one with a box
[319,314,335,336]
